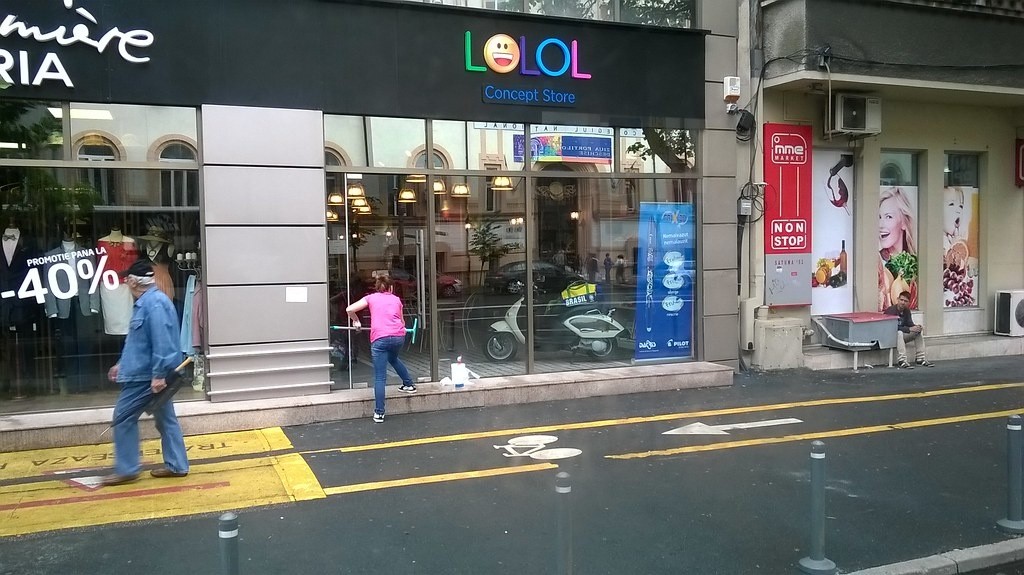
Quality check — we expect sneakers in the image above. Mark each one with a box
[898,360,915,369]
[371,412,386,424]
[396,384,419,393]
[915,357,935,367]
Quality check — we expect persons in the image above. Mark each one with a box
[943,185,965,260]
[878,187,917,260]
[103,256,191,487]
[883,290,936,369]
[552,248,629,284]
[330,275,366,366]
[0,223,176,395]
[345,276,417,424]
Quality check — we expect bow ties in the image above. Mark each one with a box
[1,234,17,241]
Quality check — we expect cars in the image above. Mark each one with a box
[361,270,416,304]
[484,260,588,296]
[436,269,464,298]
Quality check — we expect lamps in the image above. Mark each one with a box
[397,188,418,202]
[347,184,366,199]
[327,210,339,222]
[433,179,447,195]
[406,175,426,182]
[357,205,372,214]
[489,177,513,191]
[351,198,369,208]
[450,183,472,197]
[327,193,344,206]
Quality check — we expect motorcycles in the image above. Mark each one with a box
[481,272,624,363]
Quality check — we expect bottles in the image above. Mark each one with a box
[840,240,847,273]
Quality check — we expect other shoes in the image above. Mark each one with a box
[150,467,189,478]
[102,473,143,486]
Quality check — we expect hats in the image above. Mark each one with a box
[117,260,155,278]
[70,231,83,239]
[135,224,176,244]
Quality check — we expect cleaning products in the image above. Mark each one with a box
[451,355,466,389]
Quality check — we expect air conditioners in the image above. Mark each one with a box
[995,289,1024,337]
[824,92,882,137]
